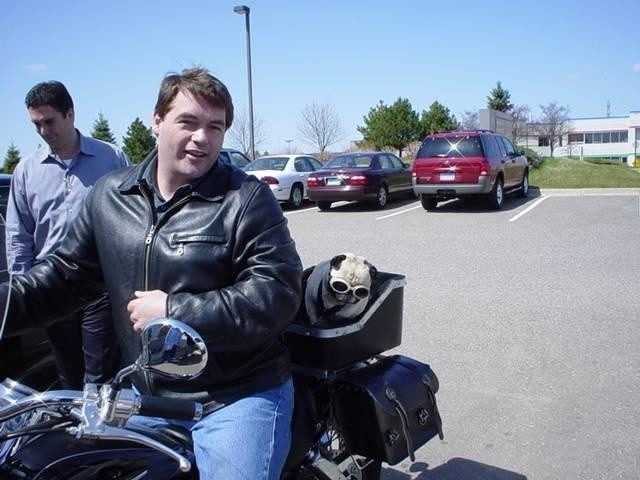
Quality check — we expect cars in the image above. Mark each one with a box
[1,172,61,387]
[240,153,324,210]
[305,150,418,210]
[220,148,253,170]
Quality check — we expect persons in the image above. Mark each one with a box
[4,80,132,389]
[0,69,304,480]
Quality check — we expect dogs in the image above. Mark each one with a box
[299,252,378,328]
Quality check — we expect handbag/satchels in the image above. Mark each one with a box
[328,355,445,466]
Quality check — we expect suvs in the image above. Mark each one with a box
[412,130,530,209]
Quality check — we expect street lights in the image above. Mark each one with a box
[232,4,256,162]
[285,139,293,153]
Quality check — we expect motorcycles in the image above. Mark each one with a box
[0,265,443,479]
